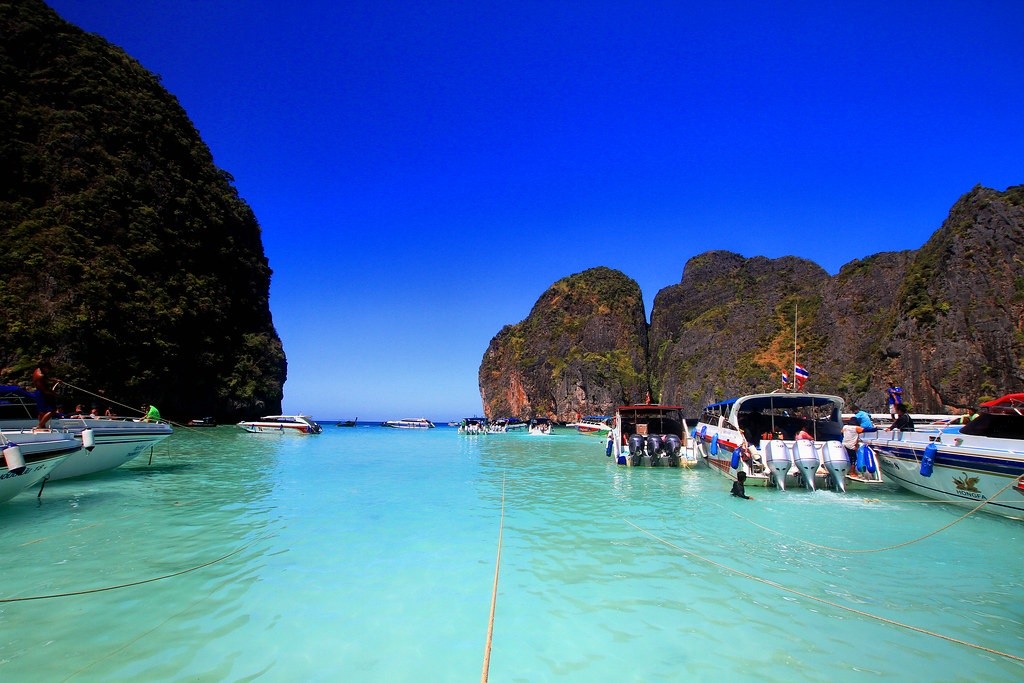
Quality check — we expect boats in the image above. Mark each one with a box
[380,422,390,427]
[694,388,851,493]
[387,418,435,429]
[0,384,174,485]
[565,416,616,433]
[182,417,219,428]
[237,412,323,434]
[489,417,527,432]
[336,417,358,427]
[0,426,95,505]
[448,416,489,434]
[528,418,552,435]
[606,399,700,468]
[820,392,1023,524]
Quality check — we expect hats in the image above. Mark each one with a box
[852,405,858,411]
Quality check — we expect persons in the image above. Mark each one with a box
[140,403,160,423]
[31,364,113,430]
[960,408,979,424]
[841,406,878,477]
[795,423,813,441]
[733,471,754,500]
[884,380,914,432]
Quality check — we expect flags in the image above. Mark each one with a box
[782,369,788,387]
[795,362,809,381]
